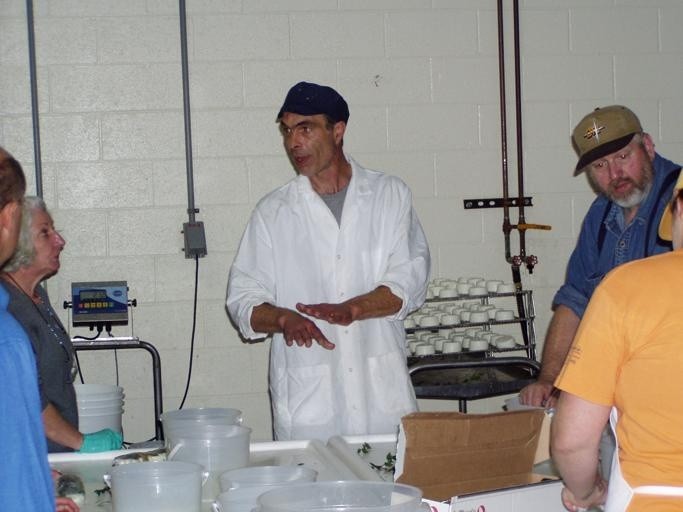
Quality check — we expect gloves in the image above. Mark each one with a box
[81,428,124,453]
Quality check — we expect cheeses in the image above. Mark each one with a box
[403,276,517,356]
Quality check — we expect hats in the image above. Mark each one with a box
[276,81,350,122]
[571,105,646,177]
[658,167,683,242]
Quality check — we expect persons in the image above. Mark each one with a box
[225,81,434,439]
[549,168,683,511]
[518,105,682,485]
[1,195,125,454]
[0,147,79,512]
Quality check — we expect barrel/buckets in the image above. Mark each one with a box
[257,478,426,512]
[73,378,125,453]
[165,425,250,512]
[210,489,266,512]
[219,466,318,486]
[159,406,240,442]
[102,457,209,512]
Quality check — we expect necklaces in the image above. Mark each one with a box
[7,272,66,341]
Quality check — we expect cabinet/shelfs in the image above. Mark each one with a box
[403,290,540,414]
